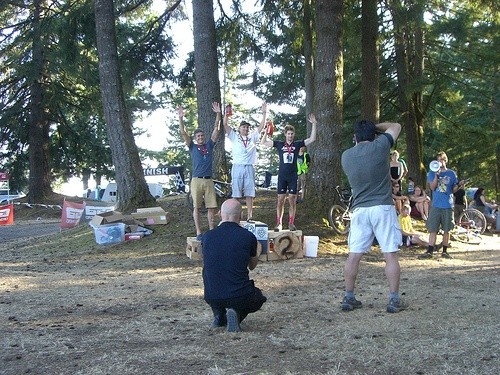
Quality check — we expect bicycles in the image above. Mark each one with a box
[327,184,354,235]
[186,175,246,213]
[454,204,487,235]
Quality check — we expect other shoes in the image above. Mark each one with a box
[196,234,203,240]
[341,296,362,310]
[274,224,282,232]
[387,297,410,313]
[289,224,297,231]
[212,314,227,328]
[442,252,452,258]
[435,242,443,251]
[226,308,243,333]
[421,214,428,220]
[418,251,432,259]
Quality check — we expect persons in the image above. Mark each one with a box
[177,101,317,241]
[341,120,410,313]
[389,150,497,259]
[202,198,268,333]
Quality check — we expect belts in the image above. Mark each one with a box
[198,176,211,179]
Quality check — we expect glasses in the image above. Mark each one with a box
[396,186,399,188]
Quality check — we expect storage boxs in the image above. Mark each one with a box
[123,215,136,225]
[131,207,172,225]
[94,222,127,246]
[89,210,123,228]
[302,235,320,258]
[125,232,144,240]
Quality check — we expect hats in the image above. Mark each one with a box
[240,121,251,126]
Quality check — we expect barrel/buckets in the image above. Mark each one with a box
[304,236,320,258]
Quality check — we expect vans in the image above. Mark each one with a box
[99,180,165,204]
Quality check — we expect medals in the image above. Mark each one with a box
[204,155,207,159]
[289,154,292,160]
[245,149,247,153]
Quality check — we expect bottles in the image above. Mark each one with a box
[266,122,274,135]
[225,104,233,116]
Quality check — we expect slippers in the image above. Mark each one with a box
[247,218,255,223]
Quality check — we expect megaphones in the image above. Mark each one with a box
[429,160,442,172]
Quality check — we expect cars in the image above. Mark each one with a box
[254,168,279,190]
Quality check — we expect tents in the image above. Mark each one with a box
[0,171,9,199]
[102,162,185,203]
